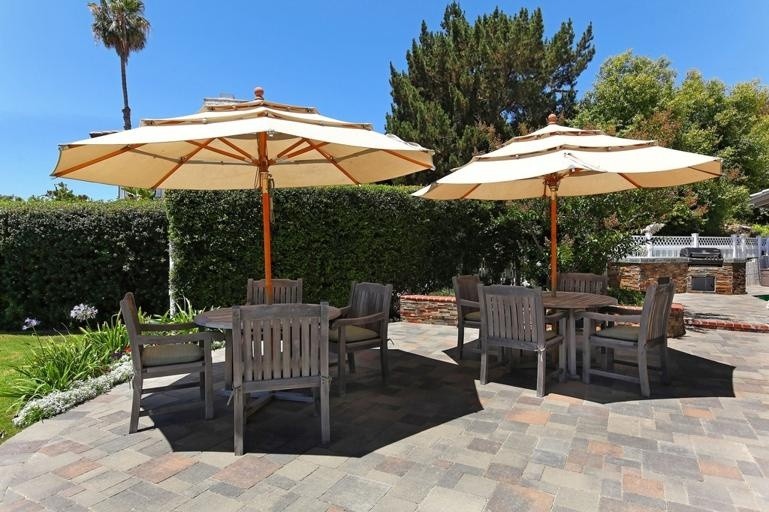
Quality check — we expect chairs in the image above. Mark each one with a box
[451,269,677,396]
[331,280,393,379]
[120,292,212,434]
[232,301,331,455]
[247,278,302,304]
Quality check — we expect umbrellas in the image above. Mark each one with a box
[49,86,438,300]
[409,113,724,302]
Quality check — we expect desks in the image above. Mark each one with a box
[193,304,342,418]
